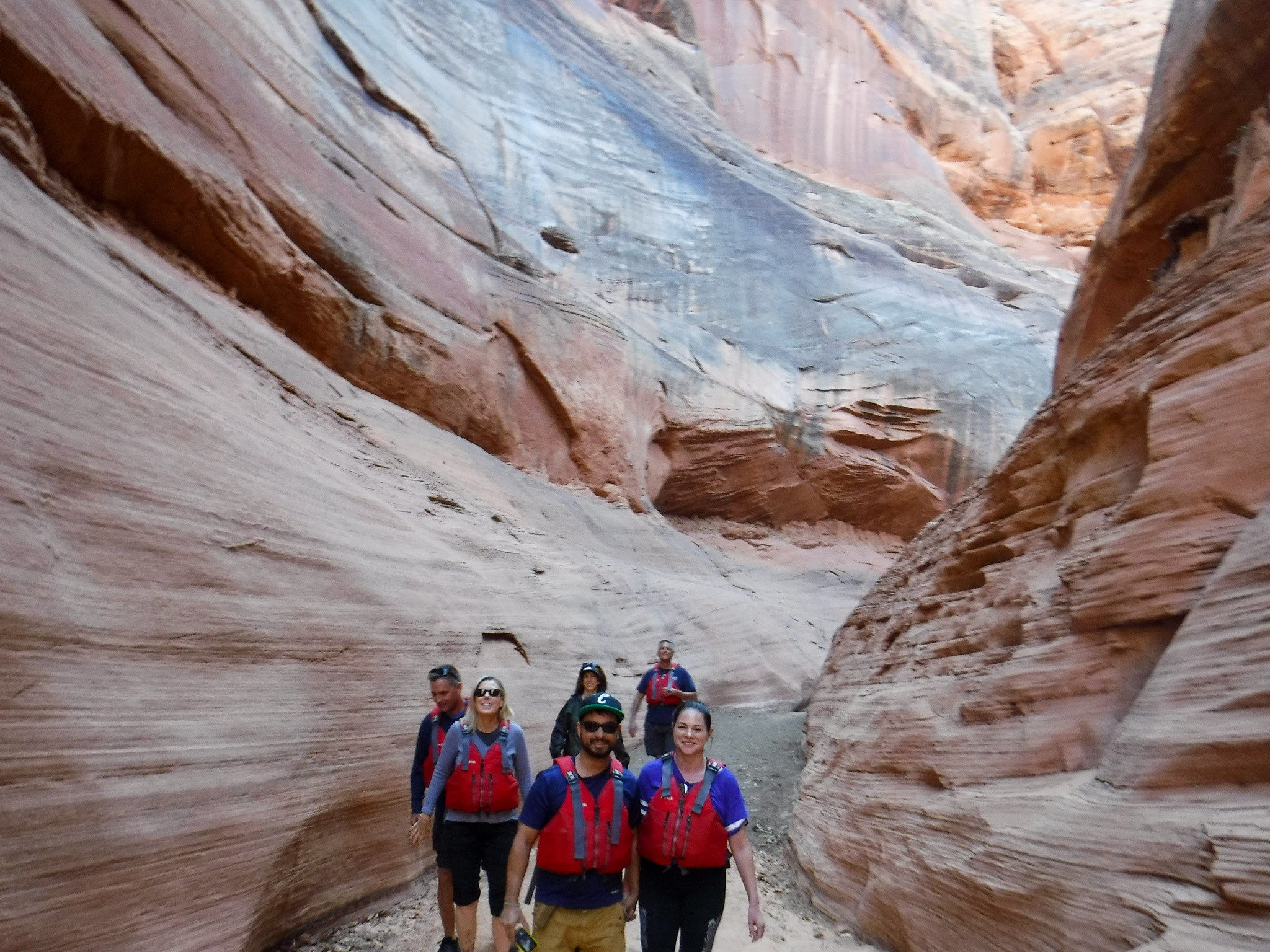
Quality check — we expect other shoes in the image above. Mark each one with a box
[509,945,518,952]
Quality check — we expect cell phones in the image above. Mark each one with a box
[514,927,538,952]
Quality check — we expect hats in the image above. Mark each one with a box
[577,692,624,724]
[583,666,600,674]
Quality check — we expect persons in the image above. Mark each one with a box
[628,639,698,759]
[498,692,637,952]
[408,663,470,952]
[549,662,631,769]
[409,676,539,952]
[622,700,766,952]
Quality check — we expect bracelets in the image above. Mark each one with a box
[420,813,432,821]
[504,901,521,907]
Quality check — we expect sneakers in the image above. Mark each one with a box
[436,935,461,952]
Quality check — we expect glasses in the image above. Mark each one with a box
[475,688,503,697]
[578,720,618,734]
[428,668,460,682]
[660,639,673,644]
